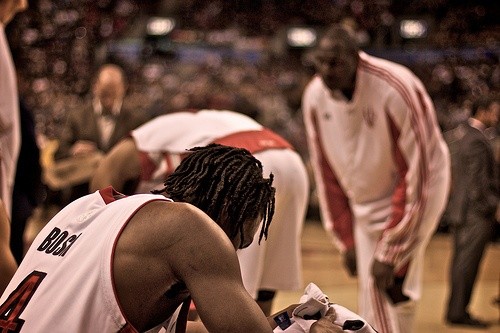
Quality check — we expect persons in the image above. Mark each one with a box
[0,0,34,296]
[22,0,498,216]
[300,19,454,333]
[1,144,361,333]
[6,0,42,272]
[95,101,312,321]
[425,61,500,331]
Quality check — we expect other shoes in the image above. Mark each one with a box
[444,310,491,330]
